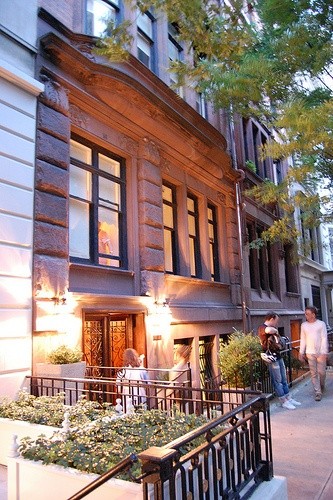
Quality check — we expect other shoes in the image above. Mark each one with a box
[320,386,324,393]
[315,394,322,401]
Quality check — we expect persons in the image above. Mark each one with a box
[260,312,302,410]
[169,345,192,385]
[299,307,329,401]
[114,348,148,411]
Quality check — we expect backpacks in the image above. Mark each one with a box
[268,333,286,360]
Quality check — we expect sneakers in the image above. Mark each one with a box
[288,397,302,406]
[282,401,296,410]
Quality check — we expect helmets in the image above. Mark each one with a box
[260,353,276,364]
